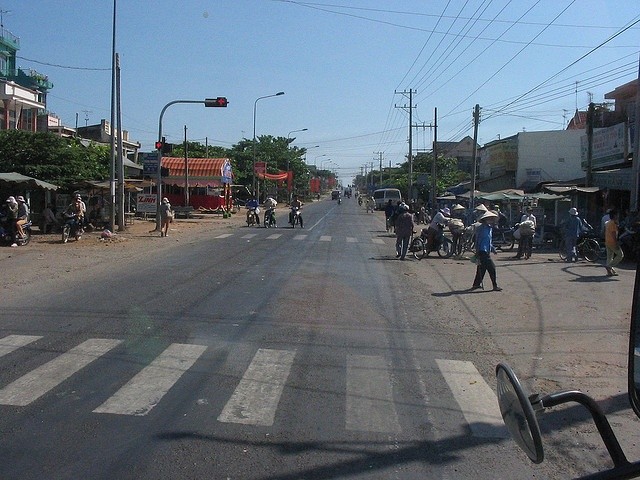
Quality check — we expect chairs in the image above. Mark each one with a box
[36,215,57,234]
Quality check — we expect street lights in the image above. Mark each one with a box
[320,158,329,172]
[314,152,326,176]
[287,126,308,171]
[301,144,318,165]
[249,91,284,202]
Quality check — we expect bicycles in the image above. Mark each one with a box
[452,230,466,258]
[395,230,424,260]
[559,232,600,262]
[467,227,514,253]
[421,228,454,258]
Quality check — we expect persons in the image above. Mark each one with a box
[62,193,87,236]
[160,198,173,237]
[263,195,278,228]
[245,196,262,227]
[472,210,503,291]
[288,194,305,229]
[515,205,538,259]
[559,207,588,263]
[394,205,415,260]
[423,206,452,258]
[5,196,18,247]
[16,195,28,239]
[40,202,61,234]
[604,209,625,277]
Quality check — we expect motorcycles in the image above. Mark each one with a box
[264,209,274,229]
[3,221,32,246]
[337,198,342,204]
[61,212,83,243]
[247,208,256,227]
[358,200,363,206]
[289,207,302,228]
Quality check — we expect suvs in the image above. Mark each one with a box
[331,190,340,200]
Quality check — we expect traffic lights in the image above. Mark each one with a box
[155,140,164,151]
[204,96,229,108]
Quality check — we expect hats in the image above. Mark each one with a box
[453,204,465,210]
[16,195,25,203]
[568,207,578,216]
[162,197,169,203]
[474,204,489,211]
[440,207,450,216]
[480,210,499,220]
[6,196,15,202]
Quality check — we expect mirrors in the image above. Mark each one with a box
[497,362,543,464]
[628,259,640,419]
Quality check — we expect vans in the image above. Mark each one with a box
[373,188,401,210]
